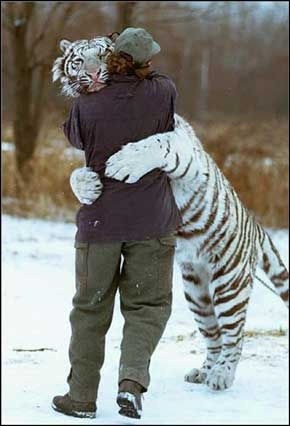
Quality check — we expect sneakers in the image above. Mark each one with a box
[50,392,99,418]
[116,378,144,420]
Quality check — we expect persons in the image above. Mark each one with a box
[51,27,183,420]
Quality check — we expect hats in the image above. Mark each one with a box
[113,26,163,66]
